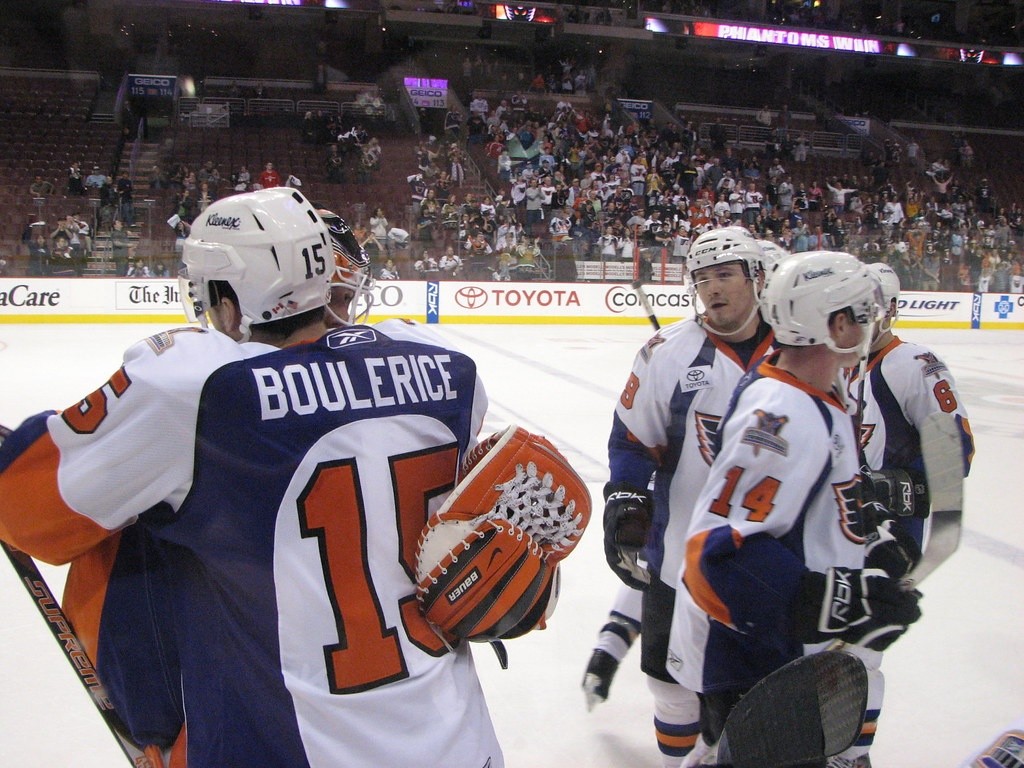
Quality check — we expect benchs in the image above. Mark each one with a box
[0,75,571,280]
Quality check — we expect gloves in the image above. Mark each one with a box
[819,565,923,653]
[859,519,921,582]
[860,465,914,520]
[603,482,652,591]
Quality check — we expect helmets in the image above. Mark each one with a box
[755,237,787,292]
[181,185,333,324]
[864,262,903,321]
[687,226,764,279]
[761,250,880,346]
[314,208,375,329]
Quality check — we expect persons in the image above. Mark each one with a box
[0,53,1023,296]
[581,224,976,768]
[0,179,511,768]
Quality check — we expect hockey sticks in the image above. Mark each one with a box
[632,277,663,334]
[2,538,153,768]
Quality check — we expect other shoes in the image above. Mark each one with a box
[582,649,618,712]
